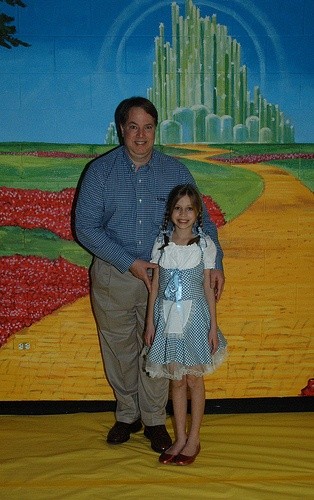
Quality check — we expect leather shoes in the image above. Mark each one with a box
[174,442,201,466]
[159,438,189,464]
[106,417,143,445]
[143,424,172,454]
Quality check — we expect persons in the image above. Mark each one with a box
[143,185,228,466]
[75,96,225,452]
